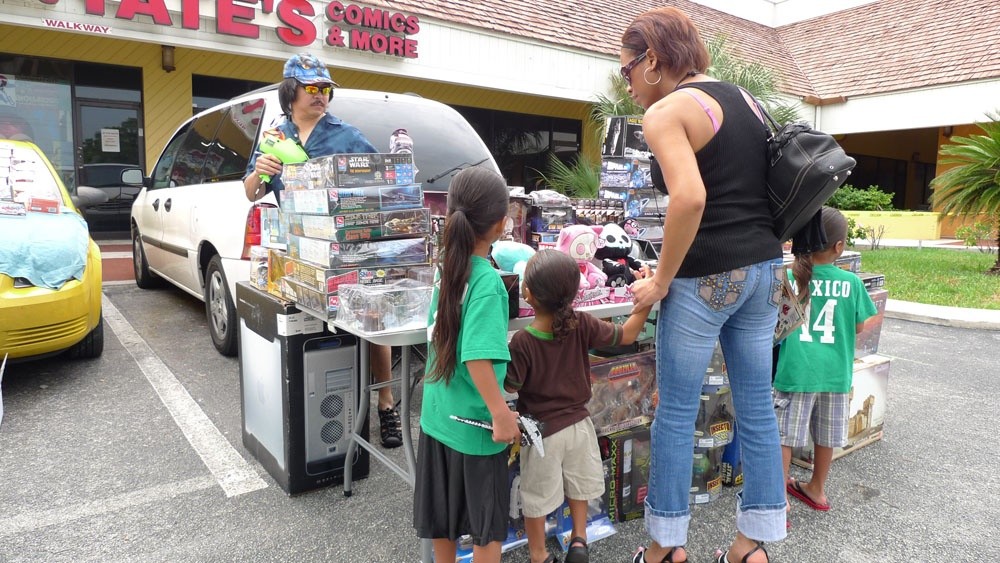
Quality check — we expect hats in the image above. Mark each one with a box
[283,53,340,87]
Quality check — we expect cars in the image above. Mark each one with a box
[1,138,104,369]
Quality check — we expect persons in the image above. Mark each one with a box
[503,248,655,562]
[242,52,382,207]
[411,166,523,562]
[621,7,787,563]
[772,206,878,510]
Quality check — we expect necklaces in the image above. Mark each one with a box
[674,71,704,86]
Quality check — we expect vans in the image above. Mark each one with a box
[70,165,144,227]
[128,79,510,357]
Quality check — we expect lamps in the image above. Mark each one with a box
[160,44,176,74]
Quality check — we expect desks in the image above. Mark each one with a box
[295,298,661,561]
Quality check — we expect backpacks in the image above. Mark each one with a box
[735,84,858,242]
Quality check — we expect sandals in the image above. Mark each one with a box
[522,536,590,563]
[631,544,689,563]
[712,537,770,563]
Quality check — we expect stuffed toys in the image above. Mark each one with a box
[595,223,646,288]
[556,224,608,292]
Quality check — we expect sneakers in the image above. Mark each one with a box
[377,404,403,448]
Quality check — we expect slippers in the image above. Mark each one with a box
[776,479,830,527]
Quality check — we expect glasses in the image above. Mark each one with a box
[621,51,647,86]
[299,85,333,95]
